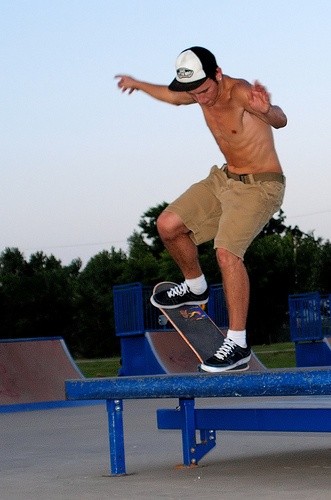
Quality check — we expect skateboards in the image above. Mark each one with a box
[153,281,250,372]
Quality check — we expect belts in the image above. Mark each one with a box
[224,166,285,184]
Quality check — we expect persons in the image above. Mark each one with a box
[114,47,287,372]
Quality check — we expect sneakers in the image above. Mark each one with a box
[201,338,252,372]
[150,281,210,308]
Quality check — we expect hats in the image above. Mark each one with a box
[168,46,217,92]
